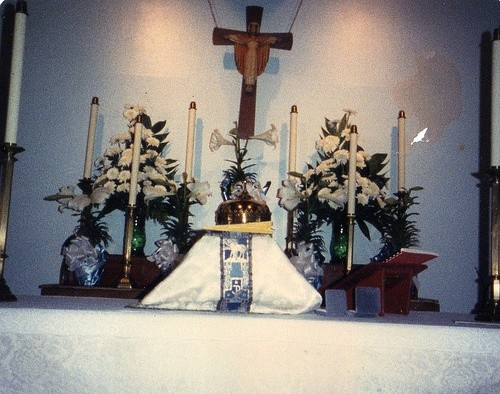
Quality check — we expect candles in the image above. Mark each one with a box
[83,96,100,177]
[185,101,197,183]
[288,105,298,181]
[489,27,499,166]
[396,109,406,193]
[349,126,359,214]
[128,114,144,204]
[4,1,30,143]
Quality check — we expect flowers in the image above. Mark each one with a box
[280,108,405,218]
[45,100,213,238]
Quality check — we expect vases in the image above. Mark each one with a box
[132,216,145,253]
[331,219,347,258]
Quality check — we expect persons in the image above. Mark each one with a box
[222,22,282,92]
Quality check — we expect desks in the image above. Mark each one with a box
[1,295,500,394]
